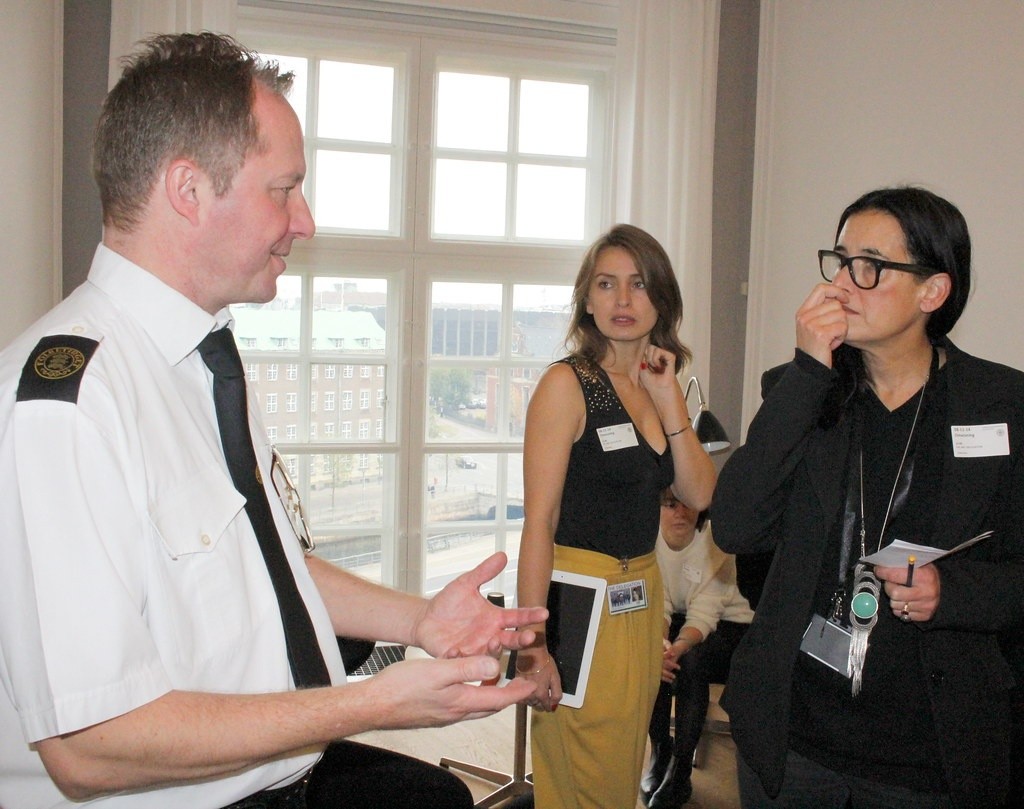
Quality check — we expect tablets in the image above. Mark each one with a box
[500,569,607,710]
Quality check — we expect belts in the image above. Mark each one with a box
[262,767,314,809]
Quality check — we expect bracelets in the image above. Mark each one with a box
[515,653,552,676]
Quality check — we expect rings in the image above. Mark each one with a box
[900,601,912,623]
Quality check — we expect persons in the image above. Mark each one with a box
[0,28,550,808]
[513,222,755,809]
[710,179,1024,808]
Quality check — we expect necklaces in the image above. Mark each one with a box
[845,342,934,698]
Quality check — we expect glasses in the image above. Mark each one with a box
[270,446,315,554]
[818,248,938,290]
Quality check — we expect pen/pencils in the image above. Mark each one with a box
[906,554,916,588]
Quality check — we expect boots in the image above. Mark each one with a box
[647,755,692,809]
[640,735,674,793]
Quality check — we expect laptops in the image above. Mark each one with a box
[346,645,409,683]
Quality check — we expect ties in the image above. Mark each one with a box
[196,329,332,691]
